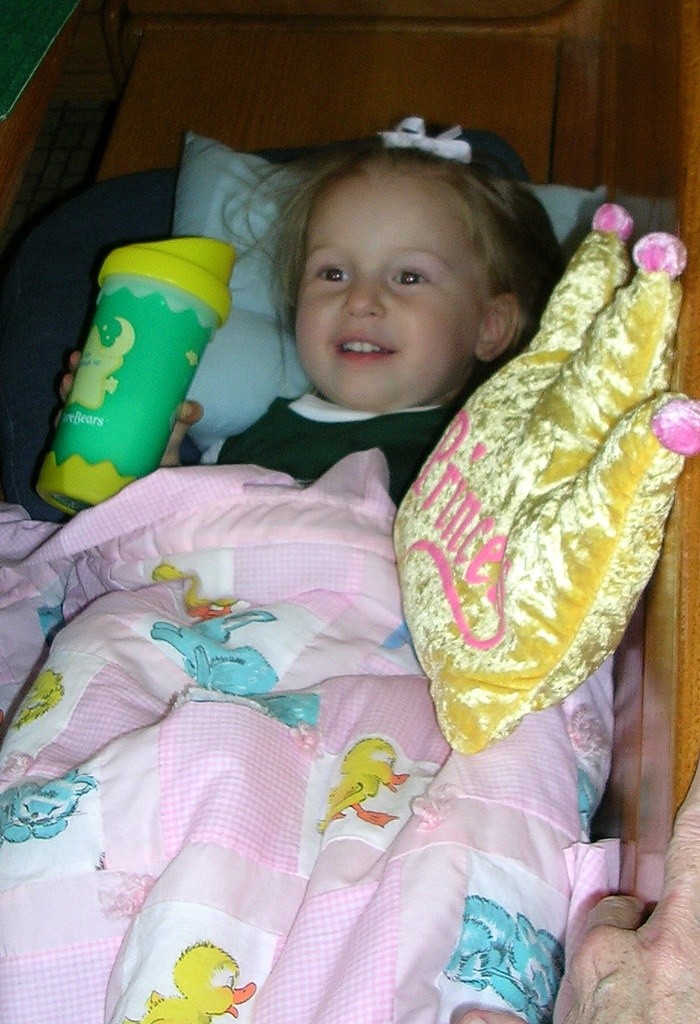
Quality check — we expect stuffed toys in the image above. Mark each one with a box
[393,203,700,754]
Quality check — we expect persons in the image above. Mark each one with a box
[58,115,565,511]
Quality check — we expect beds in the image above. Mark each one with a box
[0,0,700,1024]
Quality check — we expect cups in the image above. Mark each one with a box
[36,233,241,515]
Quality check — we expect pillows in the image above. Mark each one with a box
[169,130,608,458]
